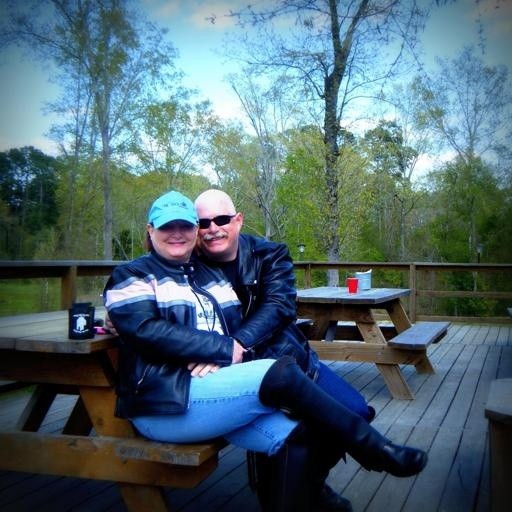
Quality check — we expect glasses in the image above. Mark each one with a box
[198,214,236,229]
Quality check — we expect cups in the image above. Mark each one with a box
[347,278,358,294]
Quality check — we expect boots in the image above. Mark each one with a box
[256,355,429,479]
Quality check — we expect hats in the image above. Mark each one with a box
[145,190,201,231]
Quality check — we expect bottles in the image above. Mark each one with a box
[69,303,95,339]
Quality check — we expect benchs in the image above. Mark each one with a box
[114,436,218,490]
[388,320,452,365]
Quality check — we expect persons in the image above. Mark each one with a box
[101,190,428,512]
[105,190,376,512]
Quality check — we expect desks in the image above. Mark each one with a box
[294,287,437,400]
[1,304,167,512]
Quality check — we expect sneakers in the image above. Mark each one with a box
[301,483,352,509]
